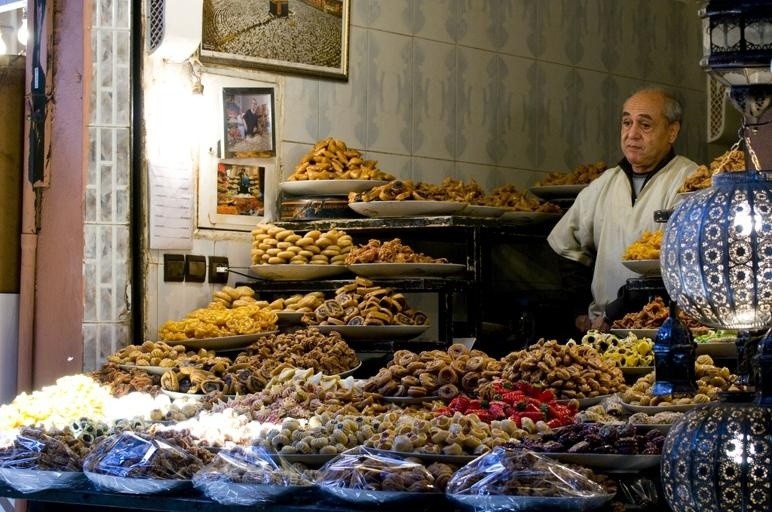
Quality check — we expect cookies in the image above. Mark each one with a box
[316,277,432,327]
[213,286,324,312]
[251,222,354,266]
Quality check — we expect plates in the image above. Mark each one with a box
[0,179,743,511]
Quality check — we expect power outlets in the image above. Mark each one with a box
[210,256,228,283]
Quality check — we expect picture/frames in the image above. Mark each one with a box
[197,146,275,232]
[221,86,278,159]
[199,0,349,81]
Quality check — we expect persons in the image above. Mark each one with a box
[546,85,701,351]
[237,168,249,194]
[243,97,259,138]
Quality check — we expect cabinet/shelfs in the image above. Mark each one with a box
[235,216,503,390]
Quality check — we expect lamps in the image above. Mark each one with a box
[660,124,771,512]
